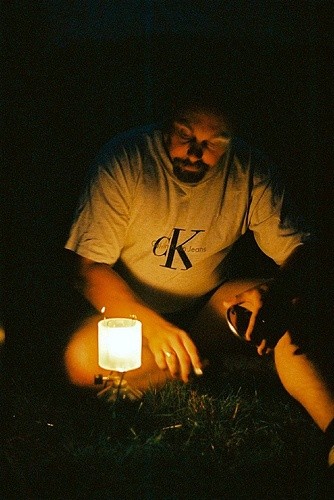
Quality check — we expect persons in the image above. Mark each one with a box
[62,80,334,467]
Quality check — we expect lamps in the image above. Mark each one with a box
[98,317,141,372]
